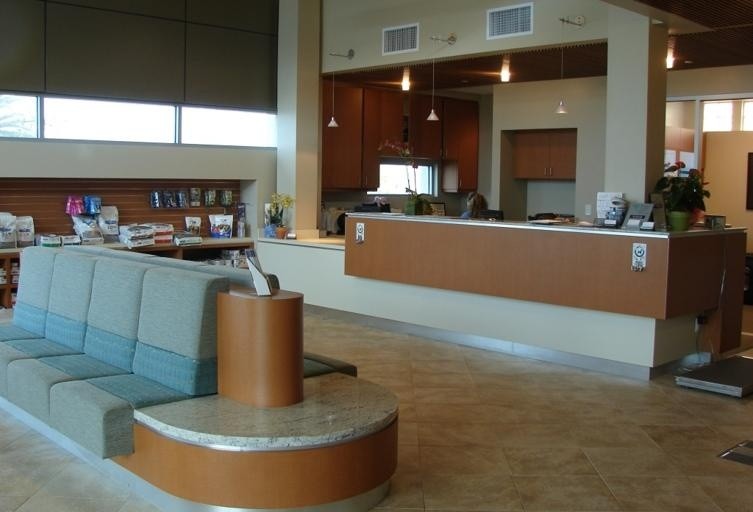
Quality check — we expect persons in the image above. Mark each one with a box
[462,192,488,218]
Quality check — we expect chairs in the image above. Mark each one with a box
[469,210,504,222]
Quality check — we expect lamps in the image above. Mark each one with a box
[501,55,511,82]
[427,39,439,120]
[555,20,569,114]
[327,56,339,127]
[401,67,410,91]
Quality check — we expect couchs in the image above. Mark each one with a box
[0,244,357,460]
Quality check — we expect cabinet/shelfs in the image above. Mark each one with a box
[322,84,480,193]
[512,132,575,179]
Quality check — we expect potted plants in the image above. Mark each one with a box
[653,160,711,232]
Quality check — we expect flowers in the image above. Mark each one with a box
[266,193,293,226]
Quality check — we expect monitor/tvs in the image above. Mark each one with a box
[429,202,446,216]
